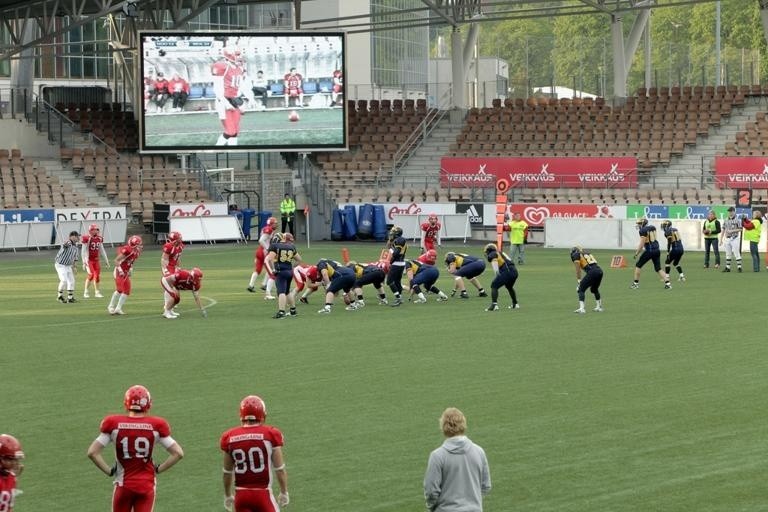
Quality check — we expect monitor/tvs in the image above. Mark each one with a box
[136,30,349,155]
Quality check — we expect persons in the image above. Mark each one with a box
[283,67,305,109]
[345,262,388,311]
[221,396,290,512]
[212,42,262,147]
[720,206,743,272]
[269,234,309,321]
[88,387,184,512]
[660,220,686,282]
[145,75,153,113]
[317,259,354,315]
[248,70,267,110]
[403,258,449,305]
[357,260,389,272]
[290,263,321,309]
[740,208,762,272]
[151,73,169,113]
[108,235,143,315]
[246,216,278,292]
[632,217,671,289]
[483,244,520,312]
[161,231,185,310]
[403,250,438,291]
[264,233,281,300]
[81,224,111,297]
[0,432,24,512]
[571,244,603,313]
[423,409,491,512]
[161,267,207,319]
[386,226,408,307]
[503,212,529,265]
[420,211,444,254]
[280,192,295,240]
[445,252,487,300]
[168,73,191,113]
[700,209,721,267]
[330,64,343,108]
[53,230,81,305]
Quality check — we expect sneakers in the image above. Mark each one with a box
[317,307,331,314]
[94,293,104,298]
[356,300,364,307]
[703,264,711,268]
[84,293,90,298]
[171,310,181,315]
[162,311,175,319]
[507,304,519,309]
[413,299,428,305]
[391,295,404,307]
[737,268,742,272]
[660,277,670,282]
[108,305,116,314]
[436,295,449,302]
[664,283,673,291]
[677,276,686,283]
[346,303,357,311]
[630,282,639,289]
[592,307,603,312]
[573,308,586,314]
[722,268,731,272]
[350,302,357,307]
[285,311,298,317]
[460,294,469,298]
[271,311,285,319]
[259,284,267,291]
[378,299,389,306]
[115,308,126,316]
[476,292,487,298]
[66,297,77,304]
[264,294,276,301]
[247,285,257,293]
[715,264,721,268]
[299,297,309,305]
[56,297,67,304]
[484,304,500,311]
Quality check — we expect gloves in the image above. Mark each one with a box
[152,463,161,475]
[222,495,235,512]
[275,492,290,508]
[272,269,279,277]
[110,462,120,477]
[450,289,455,296]
[576,286,580,293]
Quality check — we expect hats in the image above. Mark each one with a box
[727,207,735,211]
[69,231,81,236]
[157,72,164,77]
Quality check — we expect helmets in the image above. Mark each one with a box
[124,385,153,414]
[661,220,672,228]
[571,246,580,254]
[127,235,143,248]
[262,228,274,234]
[0,433,23,461]
[238,395,268,424]
[636,217,648,228]
[427,249,437,260]
[167,231,182,243]
[346,261,358,266]
[223,46,243,63]
[280,232,295,242]
[89,224,100,234]
[484,244,497,255]
[444,252,454,262]
[189,268,204,283]
[389,226,403,237]
[266,217,277,226]
[429,213,438,224]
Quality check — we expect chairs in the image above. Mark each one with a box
[616,133,696,173]
[448,143,617,157]
[347,99,426,153]
[627,188,735,205]
[36,167,52,207]
[517,188,627,205]
[493,98,605,105]
[72,147,208,224]
[455,122,618,142]
[51,174,64,207]
[270,83,284,97]
[468,105,621,122]
[12,149,27,208]
[63,182,75,208]
[637,84,763,96]
[187,86,203,100]
[315,153,395,189]
[303,81,317,95]
[725,111,768,156]
[24,156,40,208]
[338,188,473,203]
[55,102,139,147]
[0,150,15,209]
[622,93,745,112]
[319,81,333,93]
[619,114,721,132]
[205,86,215,100]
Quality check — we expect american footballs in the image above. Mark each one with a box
[344,294,351,304]
[289,111,299,120]
